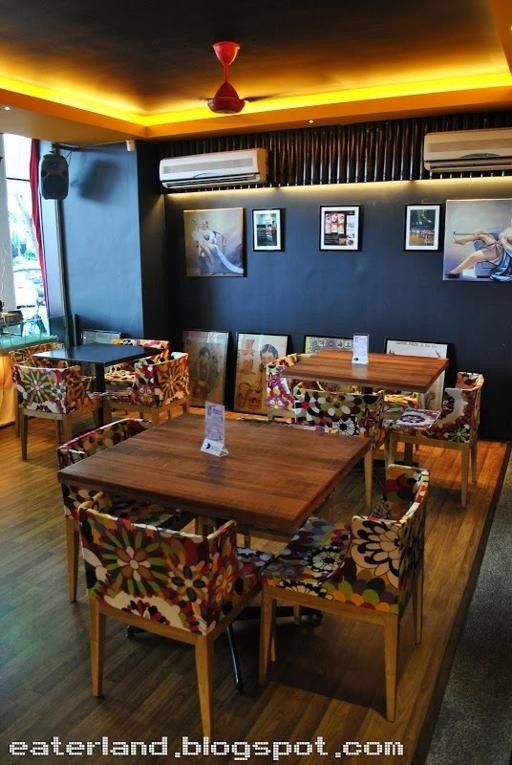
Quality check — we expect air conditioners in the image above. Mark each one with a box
[423,125,512,173]
[159,147,270,190]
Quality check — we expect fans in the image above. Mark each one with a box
[163,39,294,114]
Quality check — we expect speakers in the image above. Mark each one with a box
[40,154,70,199]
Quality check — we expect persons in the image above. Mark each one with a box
[445,224,512,281]
[255,342,280,373]
[193,346,213,389]
[192,219,219,276]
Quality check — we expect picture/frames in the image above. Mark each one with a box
[250,206,284,251]
[402,202,441,252]
[318,204,361,251]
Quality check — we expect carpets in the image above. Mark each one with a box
[411,441,512,765]
